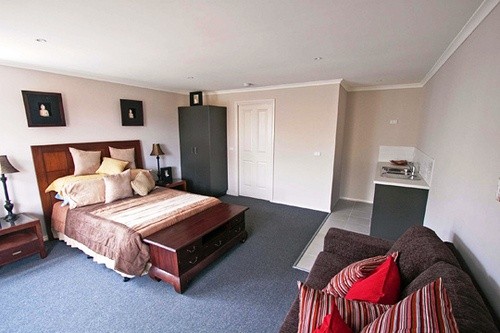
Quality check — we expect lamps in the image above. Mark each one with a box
[0,155,20,222]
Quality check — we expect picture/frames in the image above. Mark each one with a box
[22,91,66,127]
[120,99,143,126]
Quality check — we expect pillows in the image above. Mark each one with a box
[296,253,458,333]
[45,146,156,208]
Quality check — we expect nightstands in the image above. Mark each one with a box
[158,178,186,191]
[0,212,46,267]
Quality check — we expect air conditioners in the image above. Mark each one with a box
[150,144,165,184]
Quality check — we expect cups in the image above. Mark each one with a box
[407,161,421,180]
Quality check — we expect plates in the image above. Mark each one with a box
[391,161,408,164]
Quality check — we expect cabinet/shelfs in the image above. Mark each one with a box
[178,106,227,198]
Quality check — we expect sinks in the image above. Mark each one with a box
[382,166,411,172]
[380,173,420,180]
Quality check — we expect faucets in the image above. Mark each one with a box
[407,161,418,177]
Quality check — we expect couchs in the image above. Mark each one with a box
[277,224,499,333]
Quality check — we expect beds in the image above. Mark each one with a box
[31,140,249,293]
